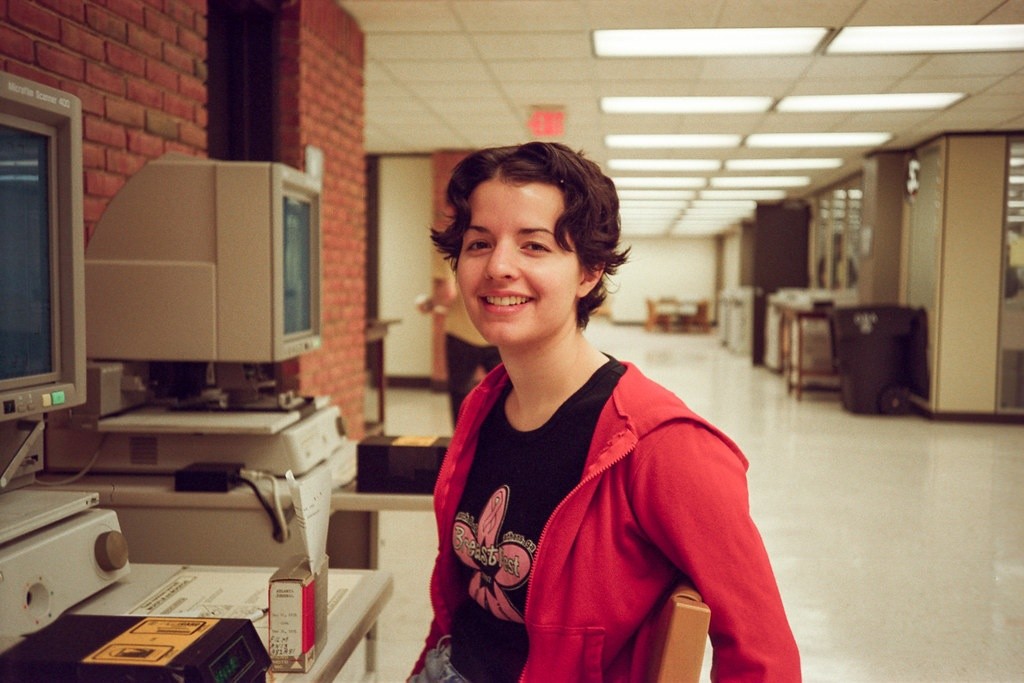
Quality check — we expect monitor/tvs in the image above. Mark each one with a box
[0,72,87,424]
[85,158,322,364]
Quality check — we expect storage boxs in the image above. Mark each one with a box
[266,553,328,673]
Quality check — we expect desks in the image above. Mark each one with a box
[364,319,404,436]
[777,303,835,403]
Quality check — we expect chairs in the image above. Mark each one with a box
[646,294,711,335]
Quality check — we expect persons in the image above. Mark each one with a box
[405,141,801,683]
